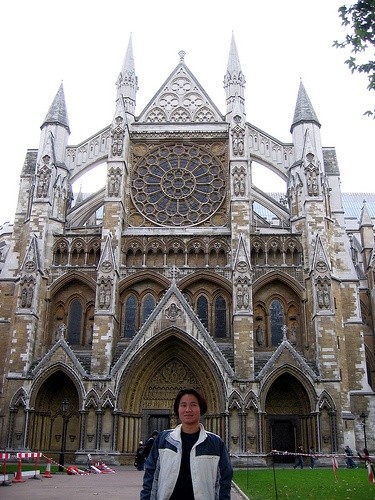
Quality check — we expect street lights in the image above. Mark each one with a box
[56,398,70,472]
[360,412,368,451]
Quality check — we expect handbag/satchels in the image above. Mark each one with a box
[134,457,138,467]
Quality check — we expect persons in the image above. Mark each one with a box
[35,175,51,200]
[140,388,234,500]
[19,287,35,307]
[236,284,250,310]
[232,135,248,197]
[108,140,124,198]
[317,287,331,309]
[292,444,375,483]
[99,286,112,307]
[134,429,164,472]
[306,175,320,197]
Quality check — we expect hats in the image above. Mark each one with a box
[139,440,143,445]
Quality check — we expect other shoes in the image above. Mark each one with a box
[344,467,348,469]
[355,467,358,469]
[309,467,312,469]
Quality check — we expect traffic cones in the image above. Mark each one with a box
[67,463,115,476]
[12,459,26,483]
[42,458,53,477]
